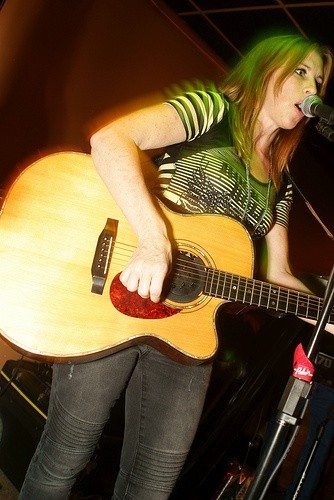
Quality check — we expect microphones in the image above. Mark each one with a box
[301,95,334,125]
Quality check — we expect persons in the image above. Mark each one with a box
[18,34,332,500]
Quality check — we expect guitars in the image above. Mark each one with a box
[0,145,334,368]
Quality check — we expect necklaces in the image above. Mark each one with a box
[240,157,272,238]
